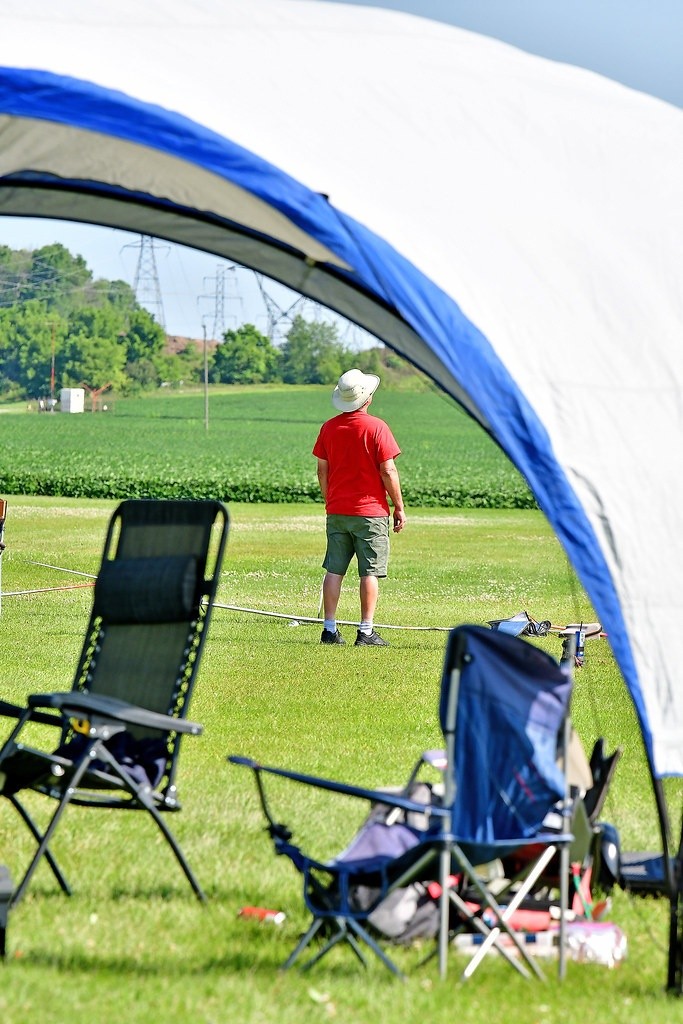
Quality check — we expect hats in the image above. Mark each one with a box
[333,370,380,412]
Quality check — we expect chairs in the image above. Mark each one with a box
[227,622,583,985]
[0,500,230,911]
[404,735,622,937]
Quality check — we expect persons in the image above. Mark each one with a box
[312,368,406,646]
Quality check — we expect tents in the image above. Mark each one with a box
[0,2,683,998]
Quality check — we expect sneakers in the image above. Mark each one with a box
[321,628,347,645]
[355,630,389,646]
